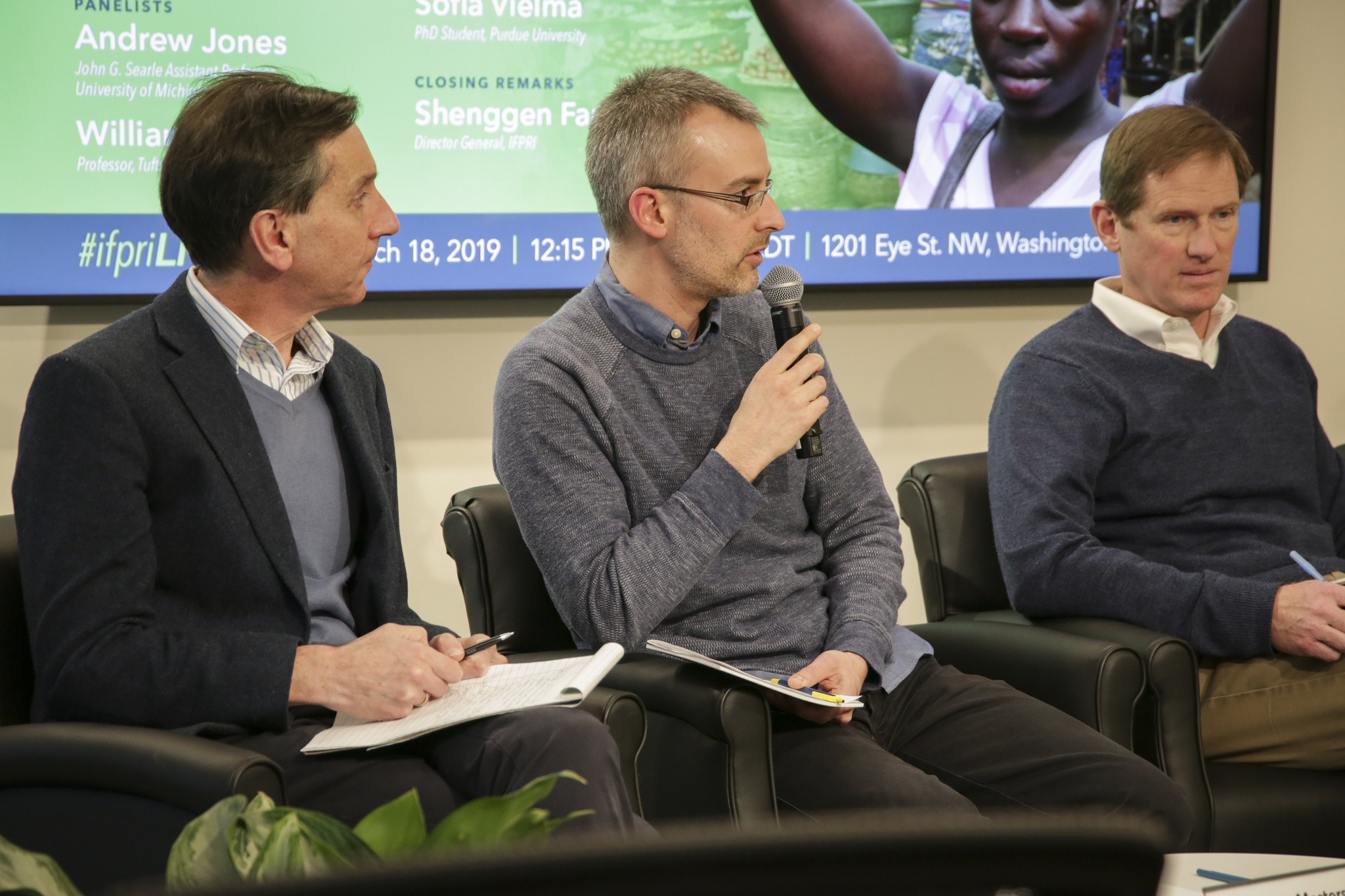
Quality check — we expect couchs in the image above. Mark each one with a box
[0,506,646,896]
[438,478,1151,821]
[896,446,1345,861]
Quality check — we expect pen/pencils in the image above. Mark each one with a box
[465,631,515,658]
[1289,550,1326,582]
[769,676,845,706]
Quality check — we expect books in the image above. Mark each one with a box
[646,639,864,708]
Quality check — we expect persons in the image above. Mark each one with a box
[492,64,1196,849]
[14,70,663,849]
[751,0,1281,209]
[987,104,1344,775]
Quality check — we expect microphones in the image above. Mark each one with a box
[760,264,822,459]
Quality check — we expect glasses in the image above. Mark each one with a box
[650,179,773,215]
